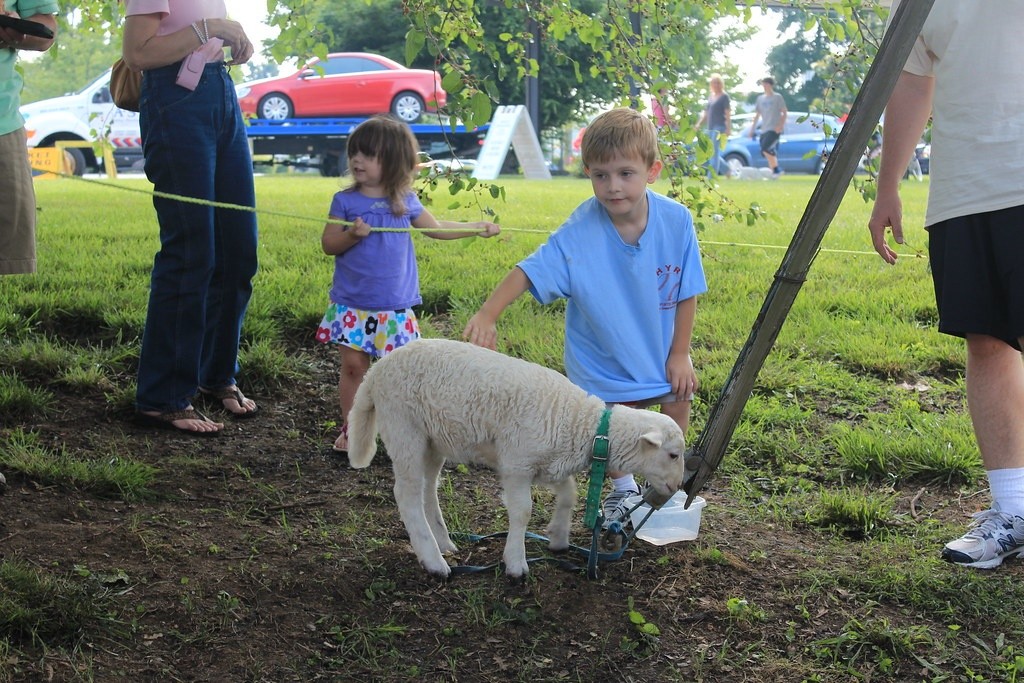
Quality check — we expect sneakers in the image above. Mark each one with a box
[939,508,1023,569]
[597,482,645,533]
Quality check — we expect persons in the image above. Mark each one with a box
[315,115,502,451]
[654,86,674,133]
[869,1,1023,569]
[121,1,260,433]
[749,78,788,181]
[1,0,61,276]
[462,107,710,531]
[694,73,735,188]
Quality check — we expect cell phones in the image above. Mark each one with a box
[0,13,54,41]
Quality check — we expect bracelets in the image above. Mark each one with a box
[192,24,205,45]
[203,17,210,43]
[193,22,207,44]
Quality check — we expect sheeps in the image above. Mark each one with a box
[347,339,686,578]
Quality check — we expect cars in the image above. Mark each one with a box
[226,48,450,125]
[677,111,859,180]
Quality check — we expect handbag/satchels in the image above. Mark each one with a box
[109,59,145,112]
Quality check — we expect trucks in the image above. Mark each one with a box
[17,62,499,179]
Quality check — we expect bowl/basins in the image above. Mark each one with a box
[624,491,708,549]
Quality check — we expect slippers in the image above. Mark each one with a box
[130,407,225,437]
[196,382,262,419]
[333,426,349,452]
